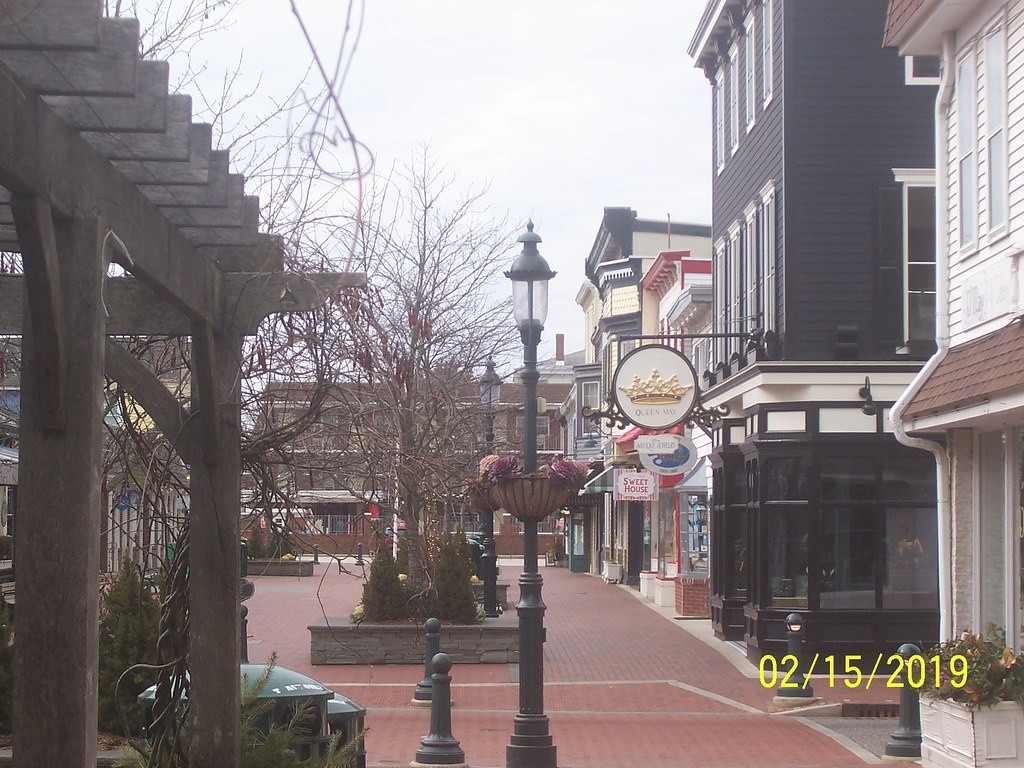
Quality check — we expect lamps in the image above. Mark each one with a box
[858,377,878,416]
[583,426,612,448]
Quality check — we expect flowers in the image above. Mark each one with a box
[899,622,1024,709]
[477,454,591,490]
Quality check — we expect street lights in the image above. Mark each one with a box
[478,355,501,618]
[502,217,560,768]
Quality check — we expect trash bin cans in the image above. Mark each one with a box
[168,542,189,574]
[240,540,247,576]
[326,692,367,768]
[602,561,623,584]
[240,536,248,577]
[137,663,336,768]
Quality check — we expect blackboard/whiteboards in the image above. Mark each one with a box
[545,551,555,563]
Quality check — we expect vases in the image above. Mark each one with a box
[919,690,1024,768]
[489,475,575,522]
